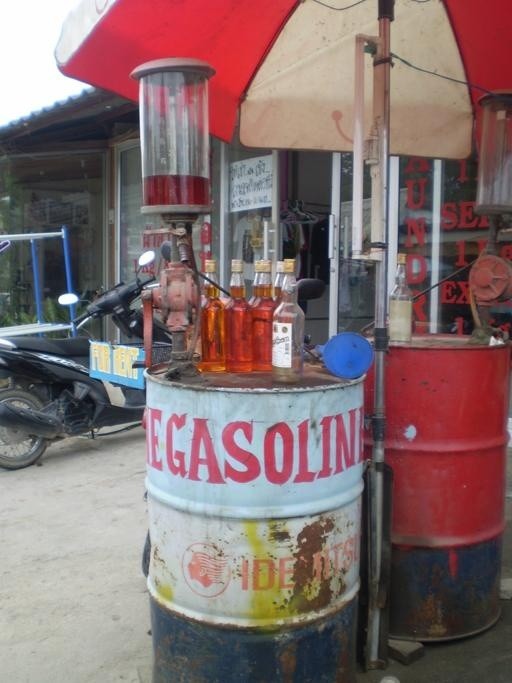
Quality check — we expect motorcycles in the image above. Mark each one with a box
[0,240,174,470]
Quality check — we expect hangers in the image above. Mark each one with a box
[269,198,323,225]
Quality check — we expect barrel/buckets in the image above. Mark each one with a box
[361,334,511,645]
[141,358,367,683]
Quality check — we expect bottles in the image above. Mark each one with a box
[198,257,307,386]
[388,252,416,347]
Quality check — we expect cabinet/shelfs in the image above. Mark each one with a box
[0,224,78,343]
